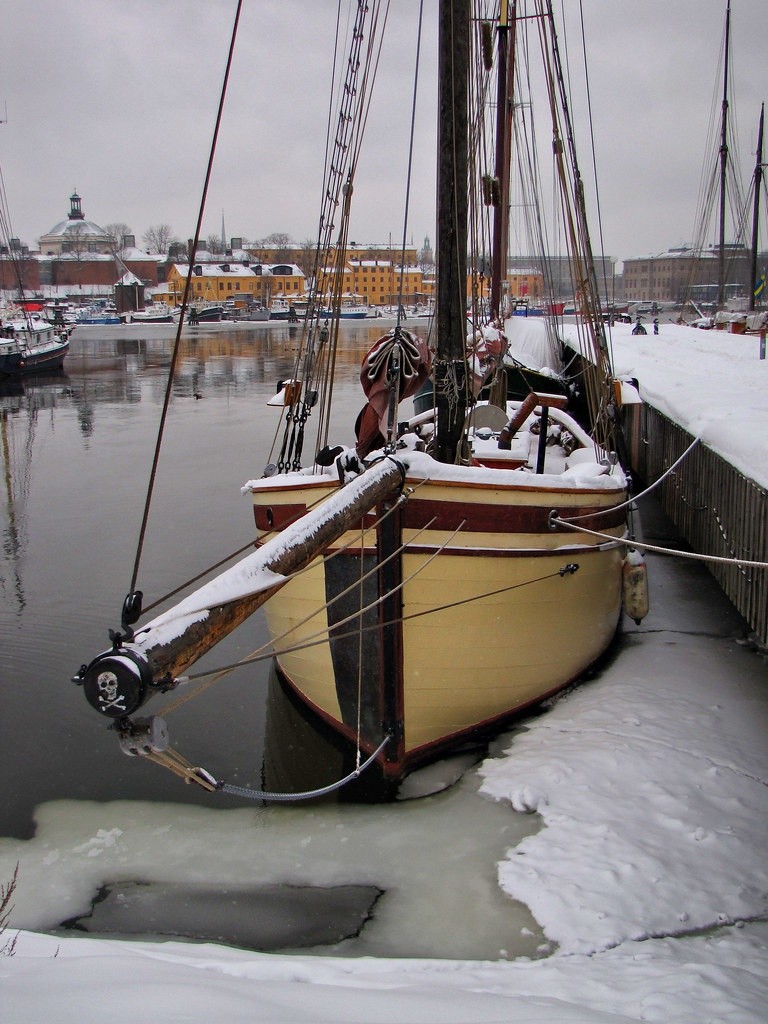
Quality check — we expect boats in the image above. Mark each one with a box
[196,282,224,322]
[269,299,290,320]
[315,306,368,319]
[292,301,314,319]
[229,307,272,321]
[130,315,174,325]
[75,315,120,325]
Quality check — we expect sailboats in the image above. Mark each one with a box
[0,97,76,378]
[669,1,768,336]
[62,0,646,811]
